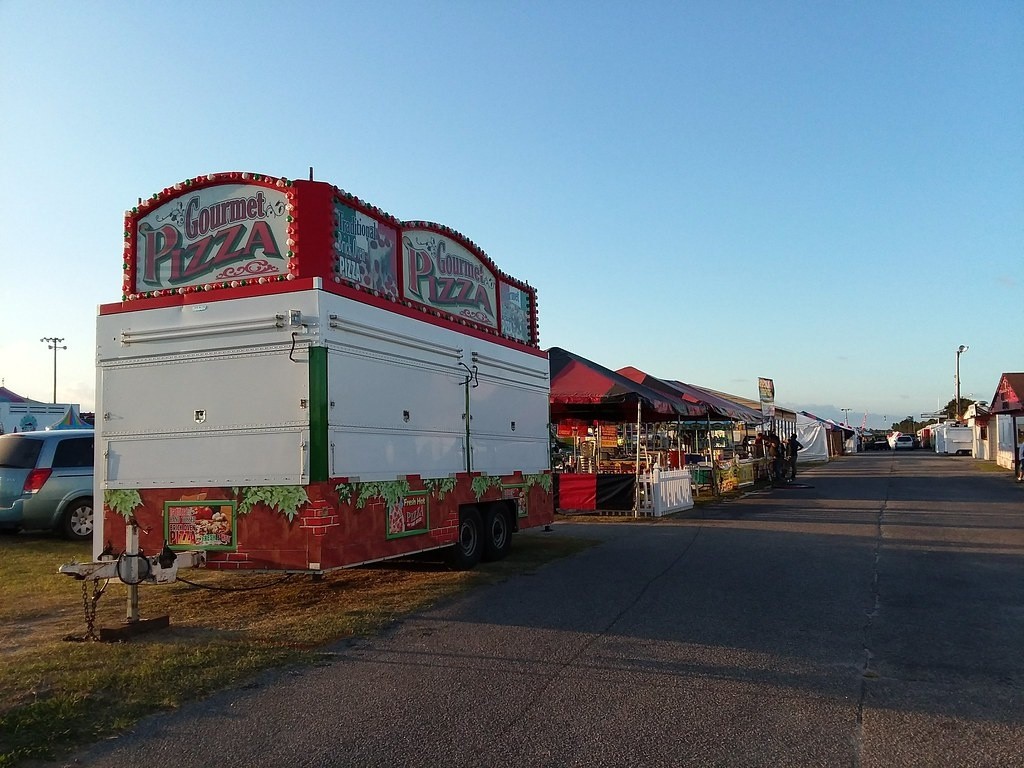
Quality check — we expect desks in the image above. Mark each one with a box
[694,467,712,491]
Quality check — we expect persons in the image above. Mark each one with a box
[755,433,804,483]
[1016,442,1024,483]
[550,437,564,457]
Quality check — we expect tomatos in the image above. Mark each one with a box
[194,507,213,519]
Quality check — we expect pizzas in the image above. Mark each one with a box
[194,519,232,537]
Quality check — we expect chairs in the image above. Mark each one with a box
[684,462,711,497]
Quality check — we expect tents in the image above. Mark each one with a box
[45,403,93,429]
[755,410,864,462]
[544,347,769,518]
